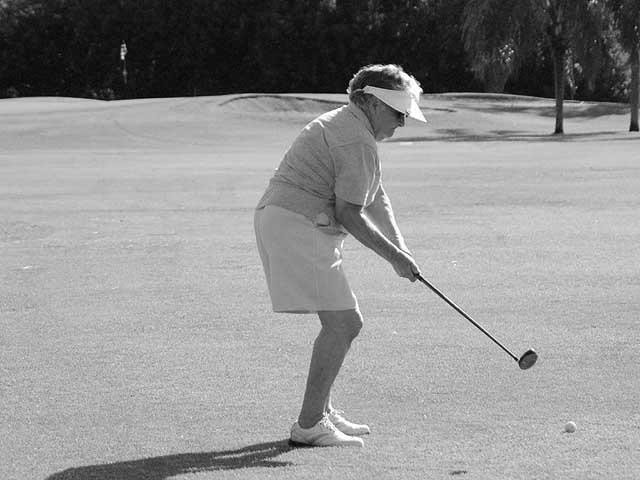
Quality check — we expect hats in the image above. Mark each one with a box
[347,86,428,123]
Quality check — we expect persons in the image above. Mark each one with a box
[253,64,428,447]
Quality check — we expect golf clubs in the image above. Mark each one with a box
[414,274,537,370]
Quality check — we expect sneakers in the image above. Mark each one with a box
[289,408,370,447]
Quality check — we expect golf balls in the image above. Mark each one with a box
[564,421,577,433]
